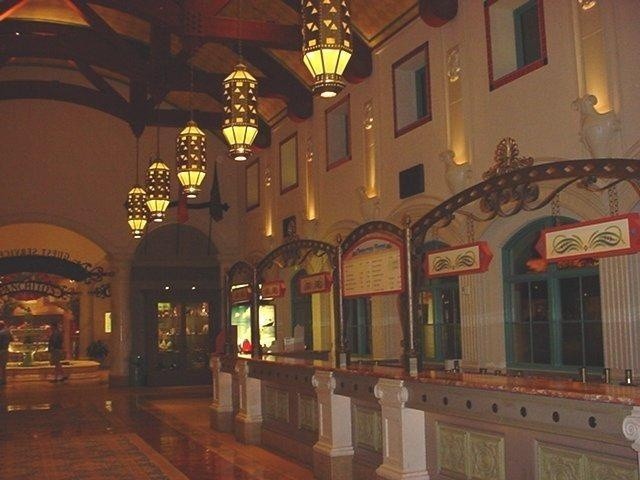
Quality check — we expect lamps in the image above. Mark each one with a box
[219,0,261,163]
[125,136,149,237]
[173,57,207,199]
[145,102,171,223]
[296,1,356,99]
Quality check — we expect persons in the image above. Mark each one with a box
[0,319,11,383]
[49,323,66,381]
